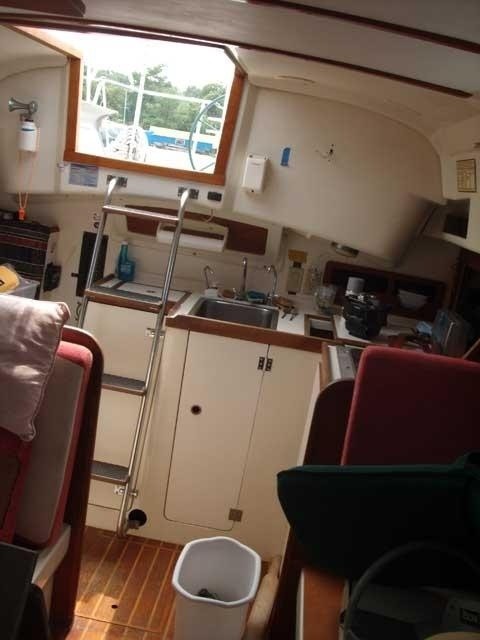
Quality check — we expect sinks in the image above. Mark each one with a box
[188,296,279,331]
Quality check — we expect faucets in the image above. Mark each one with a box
[266,265,277,297]
[201,264,213,286]
[240,257,247,297]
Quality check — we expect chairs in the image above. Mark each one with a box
[0,324,105,638]
[298,346,480,640]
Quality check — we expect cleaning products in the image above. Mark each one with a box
[116,241,135,282]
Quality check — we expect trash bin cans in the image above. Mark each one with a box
[172,536,263,639]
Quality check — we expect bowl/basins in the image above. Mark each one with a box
[398,289,427,310]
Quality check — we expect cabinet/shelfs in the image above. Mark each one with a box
[79,297,167,533]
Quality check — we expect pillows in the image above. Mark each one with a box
[2,294,71,441]
[276,451,480,579]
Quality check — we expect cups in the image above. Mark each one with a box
[347,277,366,297]
